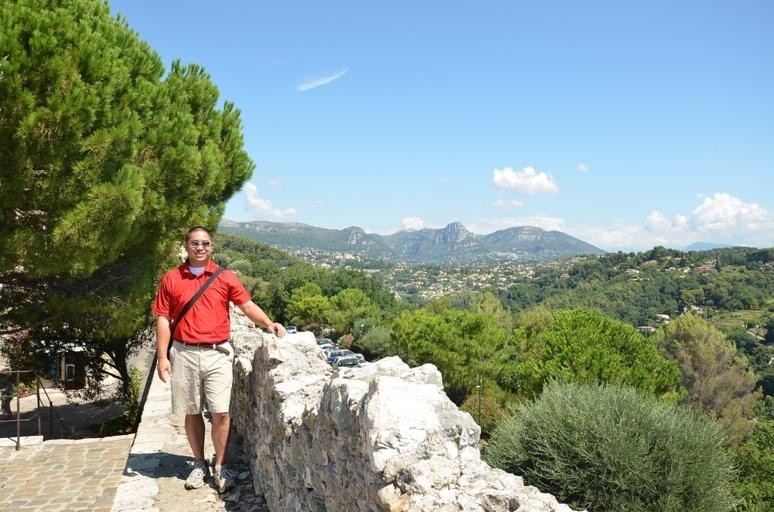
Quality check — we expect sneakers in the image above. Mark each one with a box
[212,463,236,493]
[184,460,211,489]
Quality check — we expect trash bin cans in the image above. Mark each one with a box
[44,340,87,391]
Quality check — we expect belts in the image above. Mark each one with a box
[173,337,231,354]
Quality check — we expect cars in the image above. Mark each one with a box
[317,338,372,369]
[285,326,297,334]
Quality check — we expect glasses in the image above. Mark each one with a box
[190,240,211,248]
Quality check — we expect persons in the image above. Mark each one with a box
[150,224,288,494]
